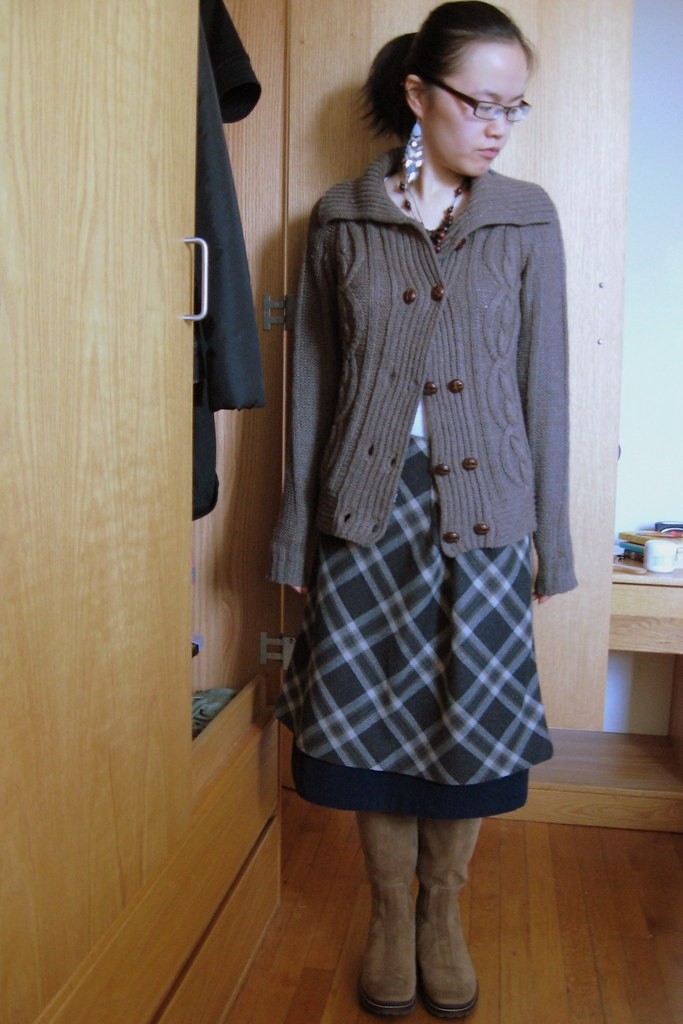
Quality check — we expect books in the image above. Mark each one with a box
[619,529,683,568]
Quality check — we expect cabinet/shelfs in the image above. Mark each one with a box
[0,0,683,1024]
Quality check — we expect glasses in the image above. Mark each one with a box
[416,72,533,123]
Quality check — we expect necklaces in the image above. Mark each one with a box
[397,181,464,254]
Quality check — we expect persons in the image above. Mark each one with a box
[268,0,578,1021]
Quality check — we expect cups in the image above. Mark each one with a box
[643,540,677,572]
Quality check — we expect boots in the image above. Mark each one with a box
[355,810,422,1015]
[418,817,478,1020]
